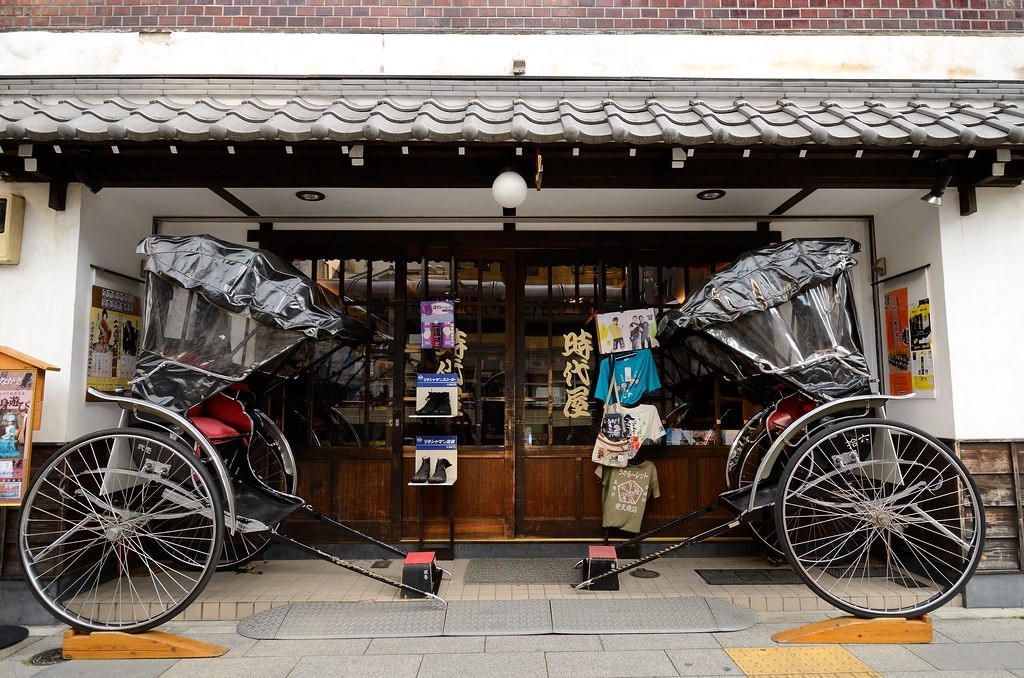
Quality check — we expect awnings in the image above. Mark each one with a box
[1,72,1024,192]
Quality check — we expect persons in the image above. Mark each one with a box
[86,289,139,378]
[608,315,652,352]
[0,370,35,478]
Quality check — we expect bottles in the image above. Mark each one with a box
[432,320,441,348]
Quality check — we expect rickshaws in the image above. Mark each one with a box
[572,236,987,619]
[14,234,453,635]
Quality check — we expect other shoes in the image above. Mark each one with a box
[411,456,431,483]
[434,392,451,414]
[416,391,434,414]
[428,457,452,482]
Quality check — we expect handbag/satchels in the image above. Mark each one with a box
[602,413,624,440]
[590,430,628,468]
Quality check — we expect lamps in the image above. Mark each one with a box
[921,154,952,206]
